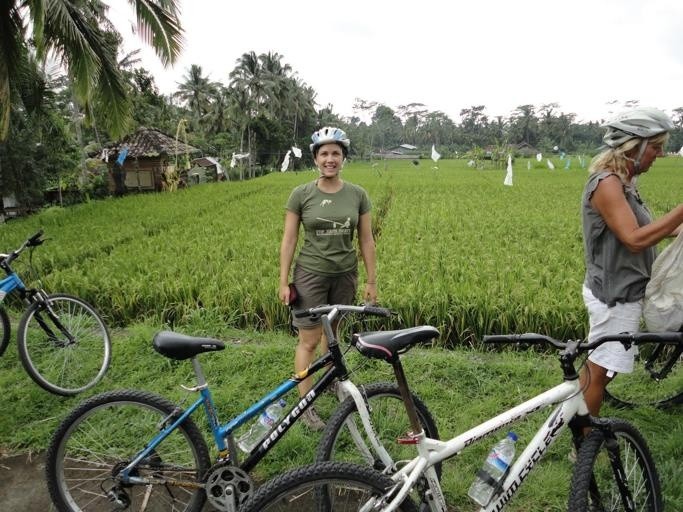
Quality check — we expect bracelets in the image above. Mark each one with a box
[366,281,376,284]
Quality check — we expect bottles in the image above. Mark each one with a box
[235,398,287,453]
[467,431,517,508]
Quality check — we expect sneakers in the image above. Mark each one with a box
[300,406,326,432]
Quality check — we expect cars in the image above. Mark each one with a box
[481,151,495,160]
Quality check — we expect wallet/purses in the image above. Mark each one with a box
[288,282,297,304]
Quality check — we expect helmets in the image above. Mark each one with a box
[600,107,674,149]
[309,126,352,159]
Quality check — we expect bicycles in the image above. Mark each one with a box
[42,301,437,512]
[0,228,112,396]
[240,321,683,511]
[600,320,682,413]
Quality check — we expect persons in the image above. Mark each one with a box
[278,125,378,433]
[565,105,682,464]
